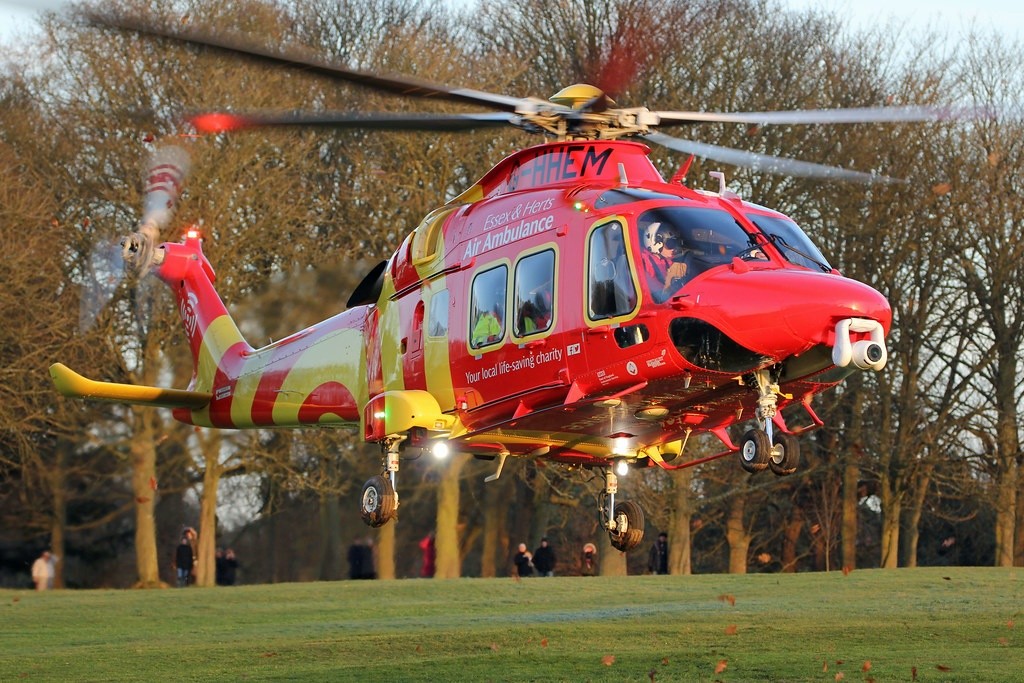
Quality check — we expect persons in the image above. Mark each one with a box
[514,538,555,577]
[31,551,58,590]
[628,221,686,311]
[419,531,433,578]
[471,287,536,349]
[346,537,378,580]
[216,547,238,585]
[173,527,197,587]
[580,543,598,576]
[648,532,667,575]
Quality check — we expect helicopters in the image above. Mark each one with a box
[6,0,1016,551]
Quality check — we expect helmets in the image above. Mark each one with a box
[642,221,681,255]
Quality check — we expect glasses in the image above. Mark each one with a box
[660,237,683,250]
